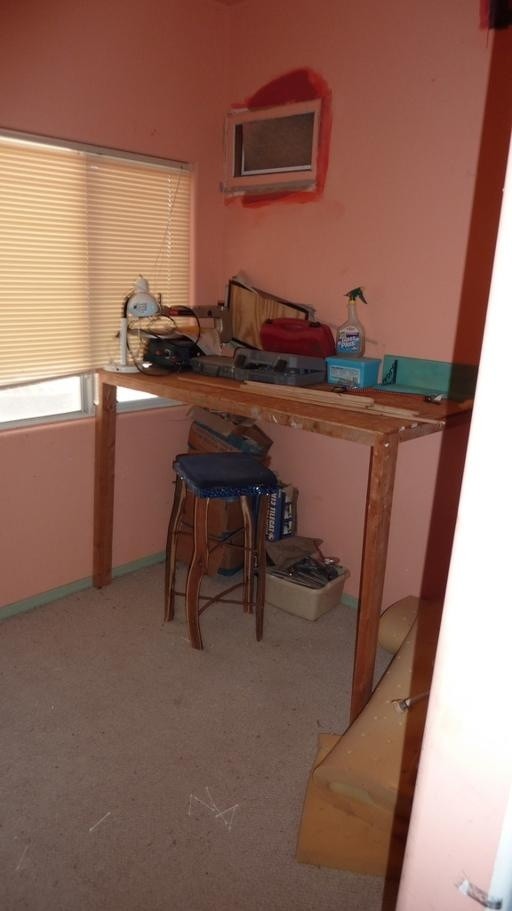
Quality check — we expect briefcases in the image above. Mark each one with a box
[259,317,336,357]
[229,343,328,387]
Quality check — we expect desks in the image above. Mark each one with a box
[91,365,447,724]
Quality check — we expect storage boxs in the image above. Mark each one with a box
[176,406,274,577]
[264,565,351,621]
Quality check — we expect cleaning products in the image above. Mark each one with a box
[336,287,367,357]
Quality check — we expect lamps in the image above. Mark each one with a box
[102,275,160,373]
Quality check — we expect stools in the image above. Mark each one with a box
[163,449,278,651]
